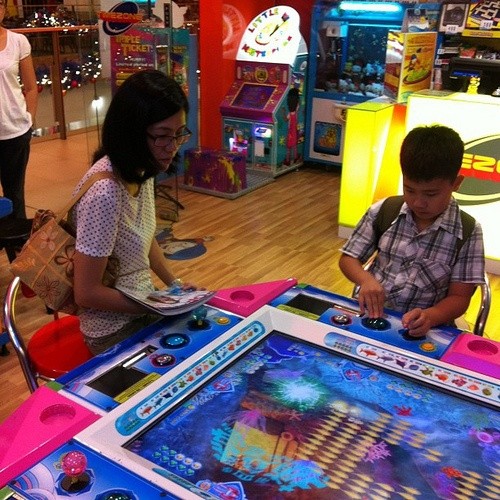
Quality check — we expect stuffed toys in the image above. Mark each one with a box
[323,59,387,97]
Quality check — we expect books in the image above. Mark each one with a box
[114,286,217,316]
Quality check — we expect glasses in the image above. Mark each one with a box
[145,129,192,149]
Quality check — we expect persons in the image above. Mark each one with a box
[339,124,483,338]
[65,69,205,356]
[0,0,41,263]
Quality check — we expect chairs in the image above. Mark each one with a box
[352,257,491,336]
[3,276,93,393]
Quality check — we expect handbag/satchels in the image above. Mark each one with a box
[8,173,121,310]
